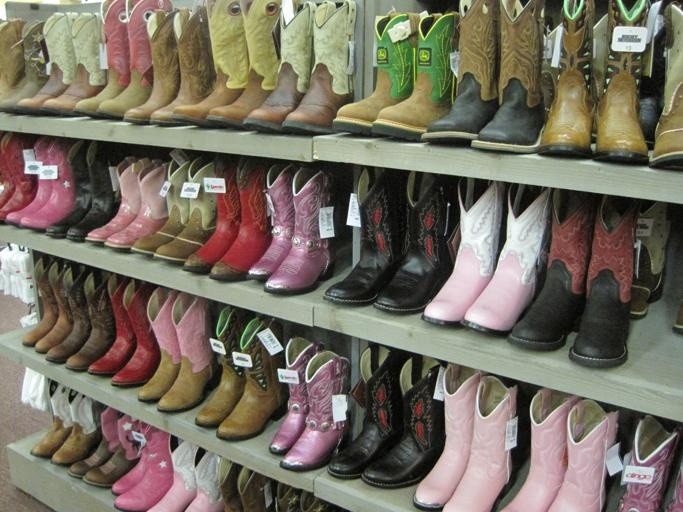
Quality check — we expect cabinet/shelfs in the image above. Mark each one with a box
[313,1,683,511]
[3,1,365,512]
